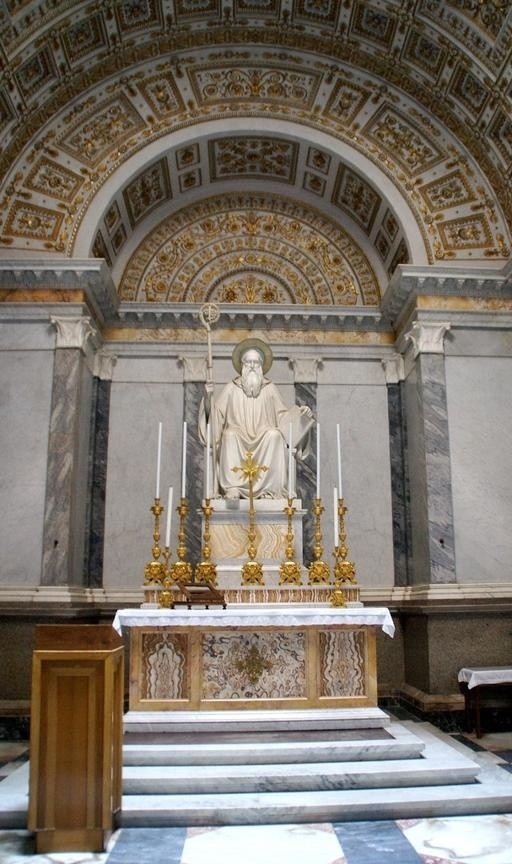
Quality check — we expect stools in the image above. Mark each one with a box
[457,666,512,737]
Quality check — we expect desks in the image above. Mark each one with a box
[112,608,396,736]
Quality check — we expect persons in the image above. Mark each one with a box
[196,337,315,499]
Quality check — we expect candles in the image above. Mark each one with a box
[155,422,162,499]
[333,487,339,547]
[289,423,292,499]
[166,488,173,547]
[181,422,187,498]
[316,423,320,499]
[336,424,342,499]
[206,424,210,499]
